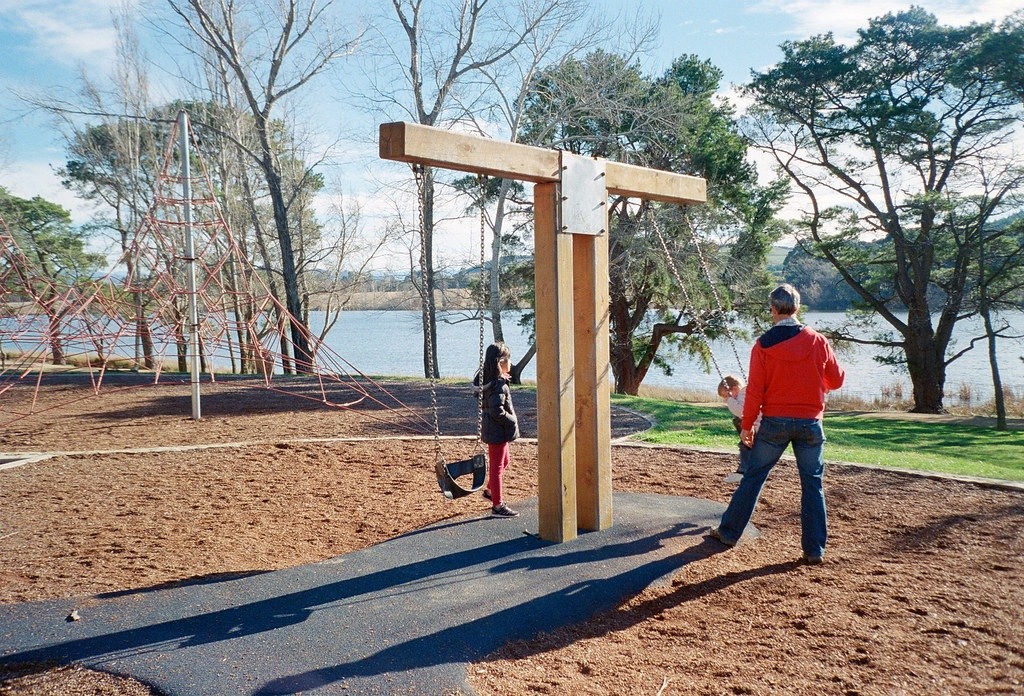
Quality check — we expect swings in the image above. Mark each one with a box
[412,161,488,499]
[642,194,764,451]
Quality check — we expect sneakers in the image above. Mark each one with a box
[803,552,823,563]
[482,490,493,503]
[491,503,519,518]
[723,473,744,484]
[710,526,736,547]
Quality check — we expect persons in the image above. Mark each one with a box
[710,284,845,563]
[717,376,763,483]
[473,342,521,517]
